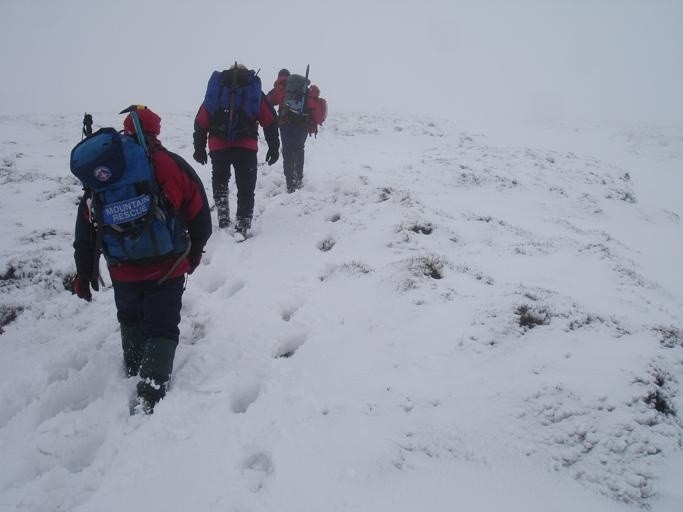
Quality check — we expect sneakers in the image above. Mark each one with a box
[233,222,251,242]
[216,214,233,229]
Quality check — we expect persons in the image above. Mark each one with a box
[70,104,212,415]
[191,63,279,243]
[263,67,309,194]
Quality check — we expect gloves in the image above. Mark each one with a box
[186,245,202,275]
[192,147,209,166]
[73,272,100,302]
[266,147,280,166]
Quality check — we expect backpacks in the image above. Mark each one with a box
[281,74,327,136]
[204,68,262,141]
[70,128,190,266]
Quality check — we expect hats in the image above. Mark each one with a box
[278,69,291,77]
[123,107,160,136]
